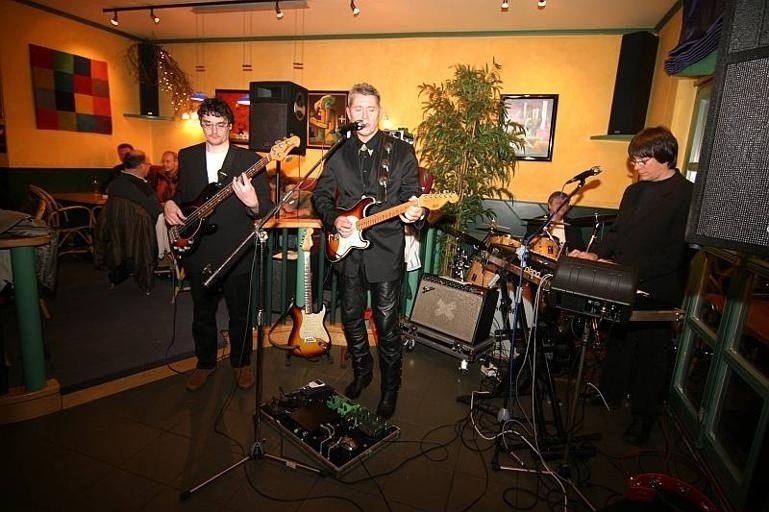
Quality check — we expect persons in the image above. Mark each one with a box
[563,123,697,448]
[277,177,317,220]
[96,142,135,193]
[152,150,179,209]
[311,82,432,420]
[516,191,586,261]
[106,148,192,293]
[162,96,270,393]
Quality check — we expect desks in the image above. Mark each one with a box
[53,193,109,206]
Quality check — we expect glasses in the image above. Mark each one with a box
[142,162,151,168]
[629,157,652,166]
[201,122,228,130]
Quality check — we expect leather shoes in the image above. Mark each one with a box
[345,379,362,400]
[623,415,652,447]
[577,389,615,406]
[186,368,217,392]
[233,366,256,390]
[377,389,397,419]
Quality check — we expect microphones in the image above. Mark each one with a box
[567,166,603,183]
[339,119,365,132]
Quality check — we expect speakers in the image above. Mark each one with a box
[608,32,659,134]
[138,43,159,116]
[409,273,499,346]
[249,81,308,156]
[684,0,769,278]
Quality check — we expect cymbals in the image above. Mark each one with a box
[474,224,509,236]
[519,217,570,226]
[567,212,622,226]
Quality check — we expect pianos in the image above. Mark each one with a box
[475,243,676,309]
[488,242,654,300]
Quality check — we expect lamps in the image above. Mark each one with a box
[349,0,361,16]
[109,8,162,28]
[275,1,284,19]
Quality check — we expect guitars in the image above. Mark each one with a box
[325,189,459,262]
[288,226,331,357]
[164,134,300,257]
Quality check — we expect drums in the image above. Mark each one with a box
[528,233,561,259]
[464,260,503,290]
[487,233,522,251]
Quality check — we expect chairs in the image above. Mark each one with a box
[26,184,104,260]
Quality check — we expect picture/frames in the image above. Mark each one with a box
[215,88,250,146]
[497,93,559,162]
[305,90,352,150]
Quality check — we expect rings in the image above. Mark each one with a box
[337,229,341,233]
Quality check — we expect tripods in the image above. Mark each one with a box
[491,321,597,512]
[457,183,584,471]
[179,134,328,500]
